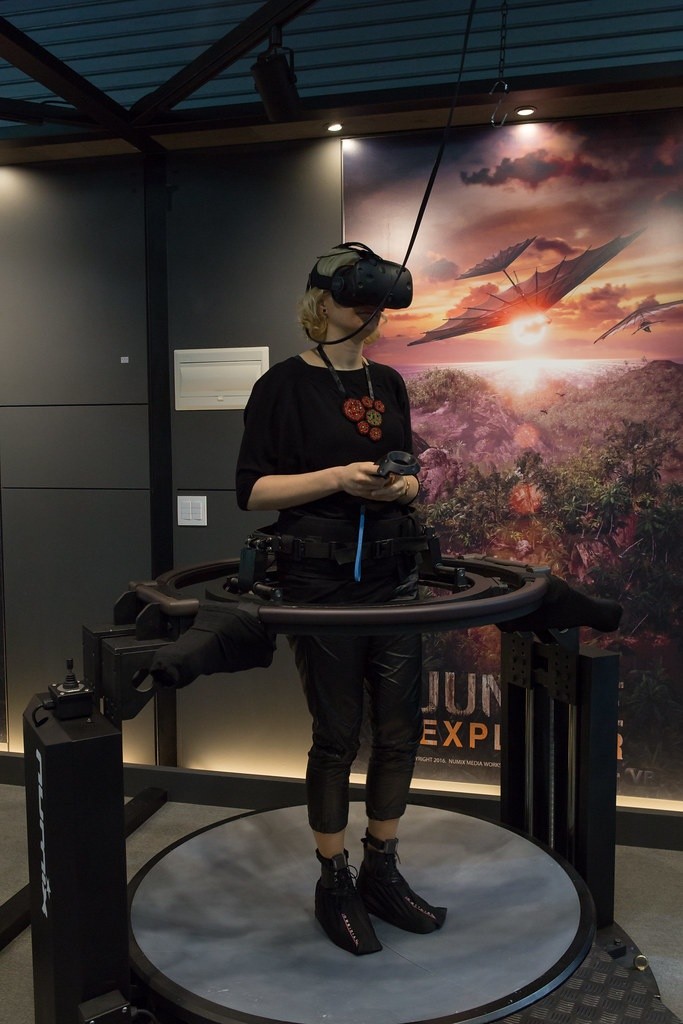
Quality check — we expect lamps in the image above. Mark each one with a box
[251,30,303,123]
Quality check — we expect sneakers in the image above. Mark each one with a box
[314,848,383,955]
[355,828,447,934]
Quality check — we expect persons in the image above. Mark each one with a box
[235,244,447,957]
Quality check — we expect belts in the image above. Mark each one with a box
[299,534,428,564]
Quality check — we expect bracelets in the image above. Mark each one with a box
[404,475,411,497]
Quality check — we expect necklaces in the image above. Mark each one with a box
[316,340,387,442]
